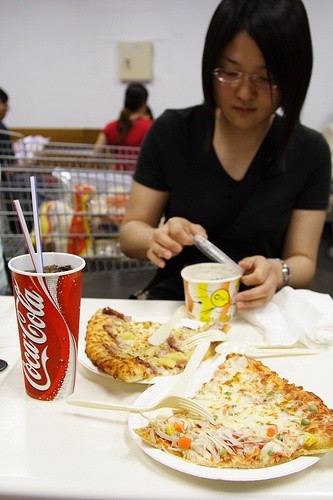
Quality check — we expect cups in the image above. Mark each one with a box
[8,252,86,404]
[181,263,243,332]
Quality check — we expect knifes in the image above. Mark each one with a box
[146,304,186,345]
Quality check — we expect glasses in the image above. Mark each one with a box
[213,68,280,89]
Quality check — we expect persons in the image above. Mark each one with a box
[0,88,34,234]
[94,83,155,171]
[119,0,331,313]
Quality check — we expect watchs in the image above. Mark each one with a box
[274,258,291,287]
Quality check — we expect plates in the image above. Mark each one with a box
[128,370,332,482]
[77,316,178,384]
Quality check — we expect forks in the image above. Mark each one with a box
[181,330,302,348]
[66,394,216,426]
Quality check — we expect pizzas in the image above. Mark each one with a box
[86,307,232,383]
[134,350,333,467]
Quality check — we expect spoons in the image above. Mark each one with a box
[215,341,321,358]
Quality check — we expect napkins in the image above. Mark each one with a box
[272,284,333,350]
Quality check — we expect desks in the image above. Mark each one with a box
[0,295,333,500]
